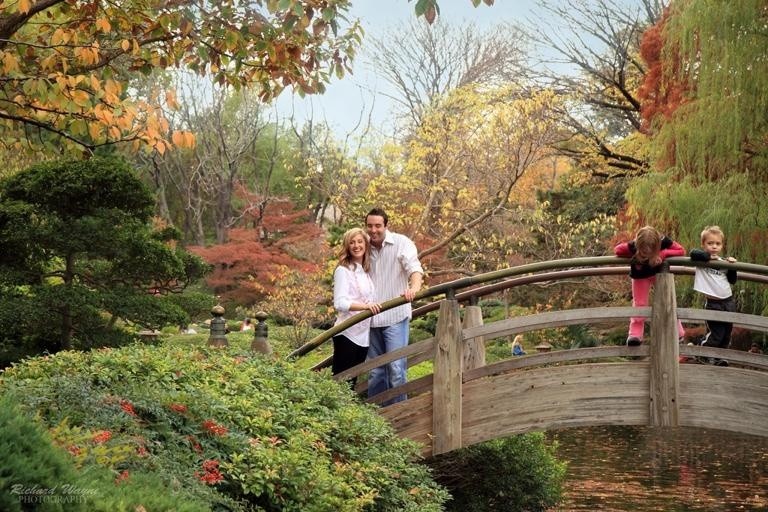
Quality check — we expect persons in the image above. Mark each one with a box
[332,228,382,403]
[689,226,737,366]
[512,335,528,355]
[748,343,764,354]
[365,208,424,407]
[240,318,254,332]
[614,226,686,359]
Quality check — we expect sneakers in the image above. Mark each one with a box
[699,356,710,365]
[627,336,641,360]
[716,359,729,367]
